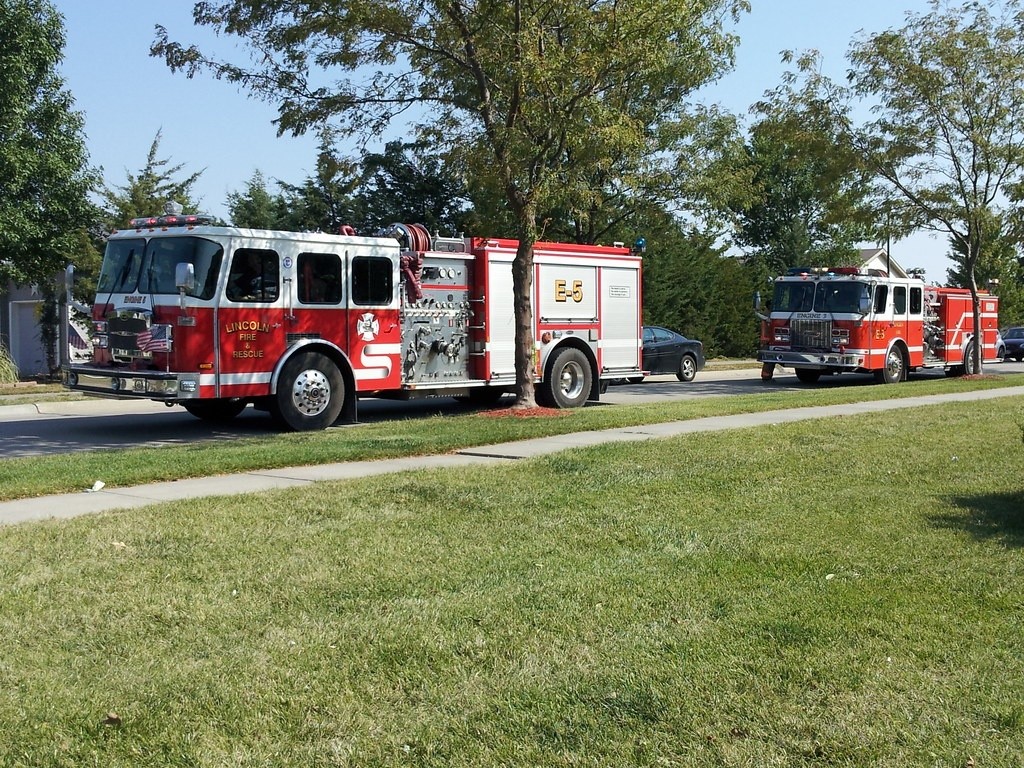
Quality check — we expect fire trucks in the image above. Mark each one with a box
[752,266,1000,385]
[57,200,652,432]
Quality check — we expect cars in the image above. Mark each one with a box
[642,326,705,382]
[995,329,1006,359]
[1001,327,1024,362]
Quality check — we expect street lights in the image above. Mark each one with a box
[883,198,892,276]
[906,268,927,281]
